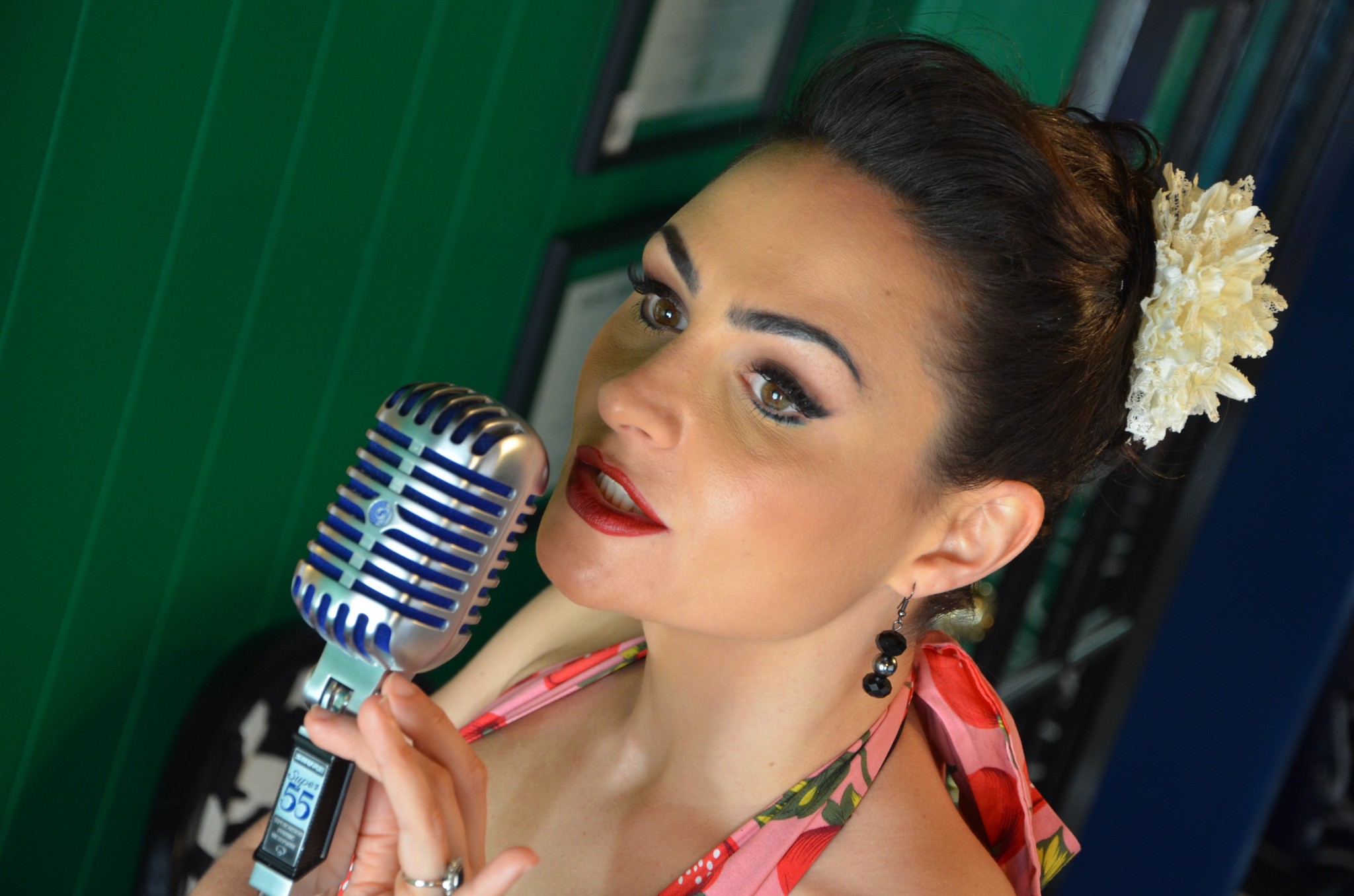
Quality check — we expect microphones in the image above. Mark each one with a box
[250,383,551,896]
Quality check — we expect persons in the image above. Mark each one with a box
[174,36,1286,896]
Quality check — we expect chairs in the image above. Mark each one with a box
[134,617,326,896]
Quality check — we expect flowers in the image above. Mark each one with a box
[1123,159,1288,452]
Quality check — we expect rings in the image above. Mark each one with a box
[400,857,467,896]
